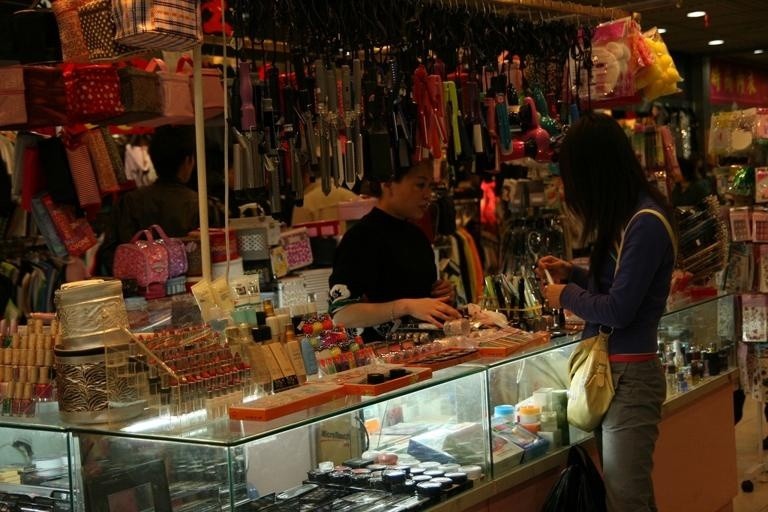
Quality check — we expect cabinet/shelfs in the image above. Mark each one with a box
[0,286,738,511]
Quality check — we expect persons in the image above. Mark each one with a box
[533,113,678,511]
[328,137,463,330]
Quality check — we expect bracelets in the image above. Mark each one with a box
[389,302,396,325]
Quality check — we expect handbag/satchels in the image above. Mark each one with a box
[0,66,67,130]
[14,0,62,65]
[541,445,606,512]
[29,194,98,257]
[51,277,144,421]
[119,65,165,122]
[176,56,226,121]
[111,0,204,52]
[113,203,314,300]
[64,64,124,124]
[145,59,193,127]
[78,0,152,64]
[567,335,615,432]
[53,0,89,62]
[12,126,136,207]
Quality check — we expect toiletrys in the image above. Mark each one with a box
[128,275,326,415]
[657,323,733,398]
[494,405,514,421]
[518,405,540,424]
[1,319,59,416]
[207,391,244,438]
